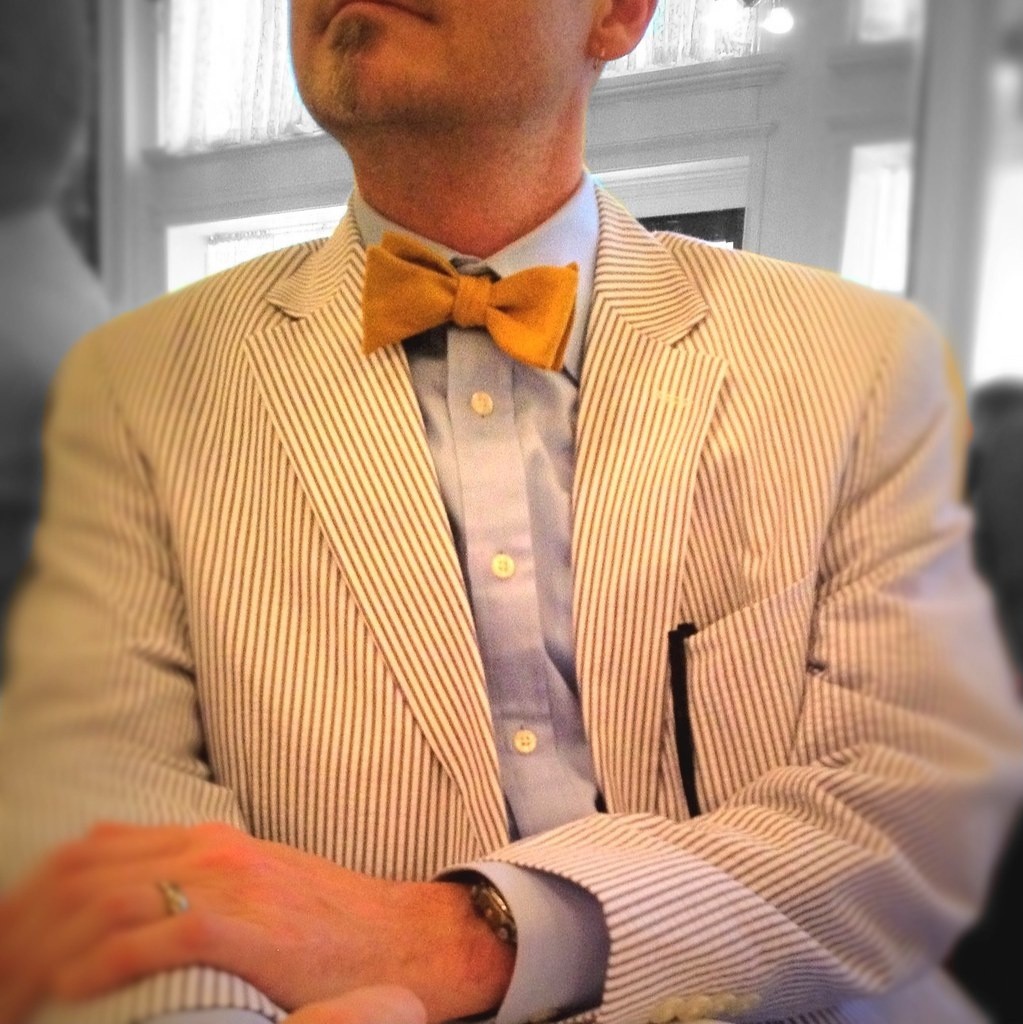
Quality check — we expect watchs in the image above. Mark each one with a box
[467,874,519,948]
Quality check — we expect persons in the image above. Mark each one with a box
[0,10,107,597]
[0,3,1022,1024]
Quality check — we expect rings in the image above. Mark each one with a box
[155,878,189,919]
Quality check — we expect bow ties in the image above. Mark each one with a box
[357,229,581,374]
